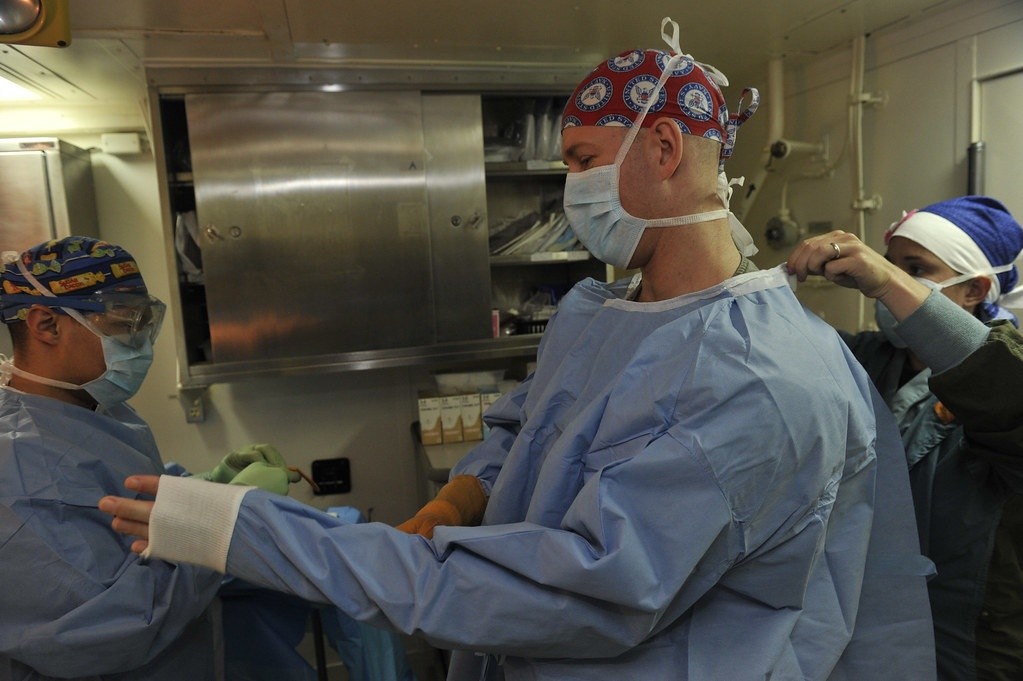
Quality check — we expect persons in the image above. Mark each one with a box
[785,197,1022,681]
[0,235,302,681]
[98,48,936,681]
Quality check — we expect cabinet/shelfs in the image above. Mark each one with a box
[144,83,617,426]
[0,139,99,360]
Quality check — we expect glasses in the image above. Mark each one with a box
[107,293,167,348]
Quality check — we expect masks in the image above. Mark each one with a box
[562,118,652,271]
[874,275,965,348]
[54,293,153,407]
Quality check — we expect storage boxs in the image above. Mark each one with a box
[416,390,503,445]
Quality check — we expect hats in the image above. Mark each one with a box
[0,235,149,324]
[559,47,760,160]
[884,194,1023,320]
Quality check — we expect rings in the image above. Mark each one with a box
[830,242,841,259]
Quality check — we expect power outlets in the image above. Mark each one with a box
[185,396,206,423]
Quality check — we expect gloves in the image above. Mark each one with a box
[228,461,302,496]
[194,443,285,483]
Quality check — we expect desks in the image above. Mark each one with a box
[409,422,488,509]
